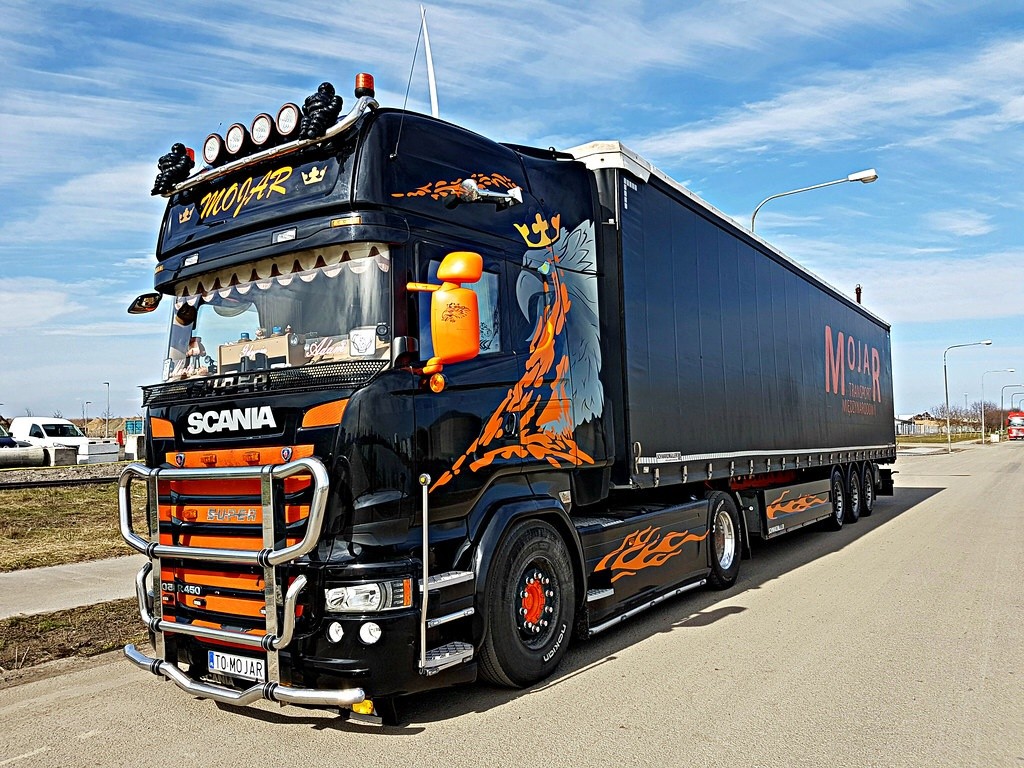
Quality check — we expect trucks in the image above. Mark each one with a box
[1006,412,1024,440]
[118,73,900,727]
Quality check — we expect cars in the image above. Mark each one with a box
[0,426,20,448]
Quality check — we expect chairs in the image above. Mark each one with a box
[34,431,43,438]
[59,427,70,436]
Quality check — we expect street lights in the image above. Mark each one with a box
[85,401,91,434]
[943,340,992,452]
[752,169,878,233]
[103,382,109,438]
[982,369,1015,444]
[964,392,968,413]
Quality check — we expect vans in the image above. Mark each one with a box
[9,416,88,447]
